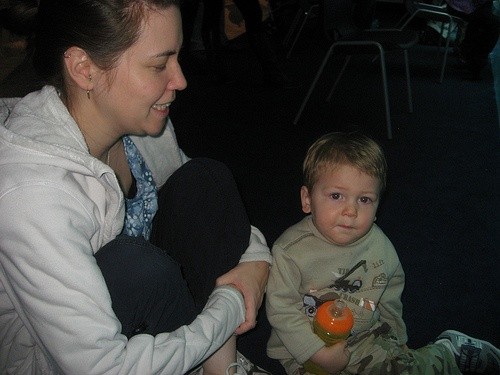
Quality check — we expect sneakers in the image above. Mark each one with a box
[438,329,497,374]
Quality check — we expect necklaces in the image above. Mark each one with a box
[106,149,110,167]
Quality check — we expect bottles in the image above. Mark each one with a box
[304,299,354,375]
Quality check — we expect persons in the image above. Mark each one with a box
[265,131,500,375]
[0,0,273,375]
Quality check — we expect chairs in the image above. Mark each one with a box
[281,0,469,134]
